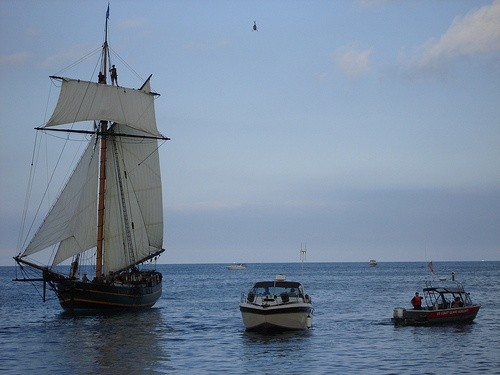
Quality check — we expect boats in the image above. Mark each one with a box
[225,263,247,270]
[239,275,315,331]
[390,271,482,325]
[369,259,377,267]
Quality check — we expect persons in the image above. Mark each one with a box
[451,296,464,308]
[410,292,423,310]
[82,274,90,283]
[91,273,109,286]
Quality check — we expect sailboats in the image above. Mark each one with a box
[10,2,171,314]
